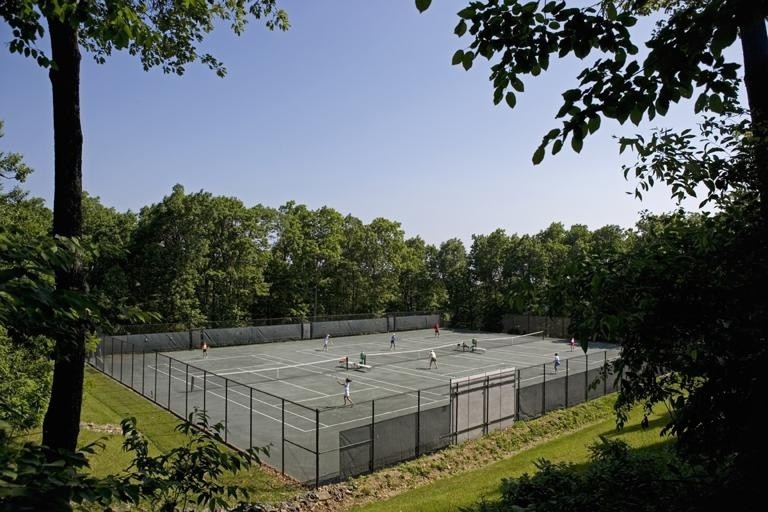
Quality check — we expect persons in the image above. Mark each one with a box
[201,340,208,359]
[552,353,560,374]
[321,334,331,350]
[432,323,440,338]
[335,377,354,408]
[427,350,437,370]
[569,337,575,352]
[388,333,397,350]
